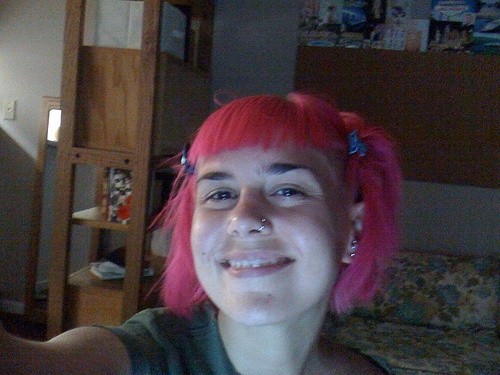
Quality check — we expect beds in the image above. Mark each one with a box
[319,248,500,375]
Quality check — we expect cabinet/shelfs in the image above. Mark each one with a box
[45,0,214,340]
